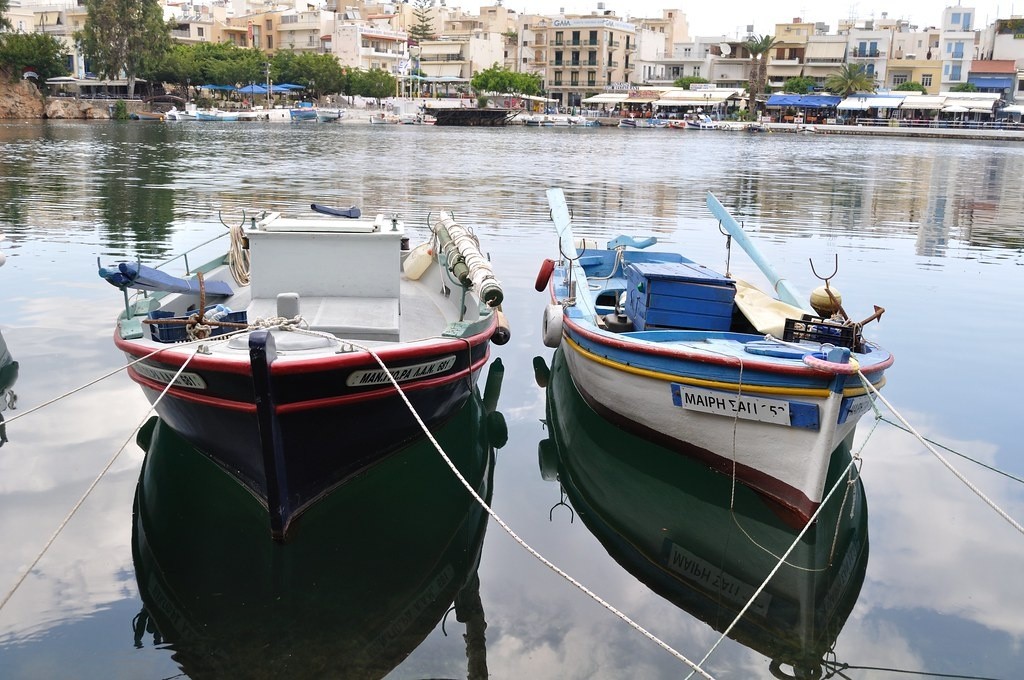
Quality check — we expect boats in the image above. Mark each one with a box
[533,344,869,680]
[519,112,768,133]
[96,204,509,543]
[130,355,508,680]
[534,185,894,527]
[132,101,436,126]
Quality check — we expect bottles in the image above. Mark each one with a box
[403,243,432,279]
[605,301,634,333]
[205,305,223,319]
[210,308,231,328]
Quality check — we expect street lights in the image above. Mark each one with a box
[258,62,269,103]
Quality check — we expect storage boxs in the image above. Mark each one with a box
[624,263,736,330]
[149,305,248,343]
[783,314,858,351]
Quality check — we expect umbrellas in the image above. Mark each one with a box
[237,84,267,106]
[258,83,289,105]
[194,84,236,102]
[277,83,306,100]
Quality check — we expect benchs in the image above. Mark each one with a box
[238,231,401,343]
[587,276,628,307]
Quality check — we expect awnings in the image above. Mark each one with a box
[1000,104,1024,115]
[940,92,1002,113]
[968,78,1012,88]
[765,94,841,107]
[901,95,947,110]
[836,94,906,111]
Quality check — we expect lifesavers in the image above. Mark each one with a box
[538,438,558,481]
[541,304,563,347]
[532,356,550,388]
[536,258,556,293]
